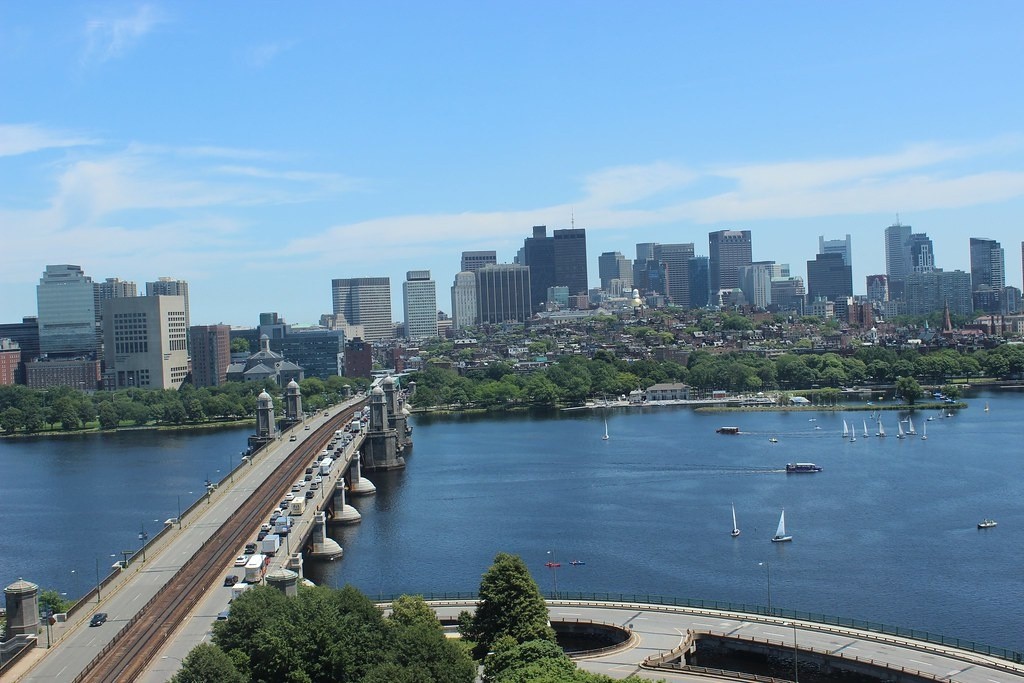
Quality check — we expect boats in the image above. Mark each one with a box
[976,518,998,528]
[544,561,561,568]
[769,437,778,443]
[567,559,586,565]
[716,427,739,434]
[785,460,824,473]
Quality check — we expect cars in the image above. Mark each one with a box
[216,404,369,626]
[89,612,107,626]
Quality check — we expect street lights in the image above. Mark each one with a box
[546,547,558,599]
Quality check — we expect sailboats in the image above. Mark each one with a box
[808,391,990,443]
[770,507,792,543]
[729,502,740,536]
[602,420,610,440]
